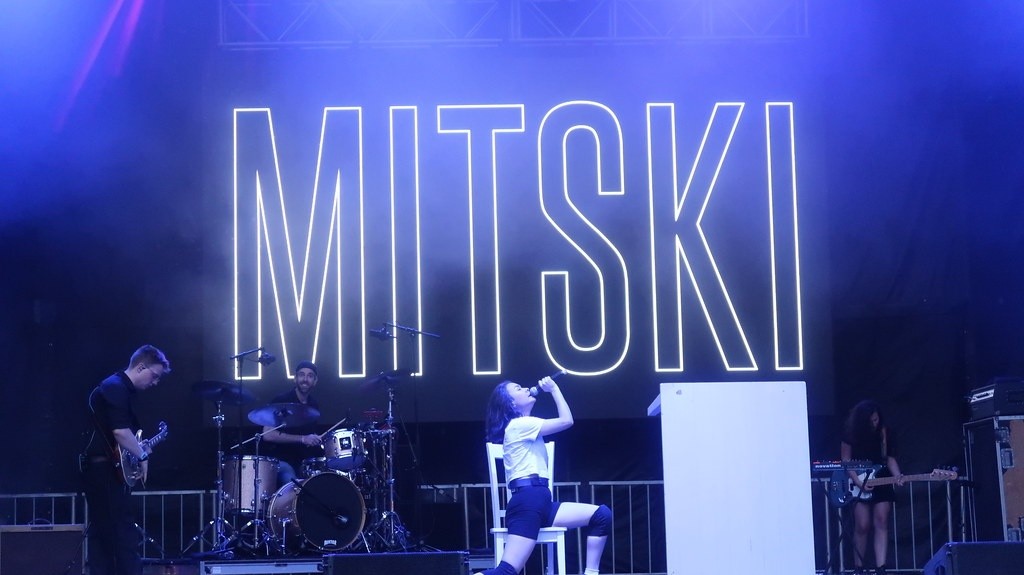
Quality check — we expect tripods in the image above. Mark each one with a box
[177,387,287,560]
[351,376,441,552]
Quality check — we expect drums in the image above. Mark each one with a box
[267,471,367,552]
[323,427,367,470]
[304,456,354,481]
[223,454,281,517]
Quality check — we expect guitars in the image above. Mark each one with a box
[114,421,168,489]
[828,463,959,508]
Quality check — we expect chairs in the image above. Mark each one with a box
[486,441,567,575]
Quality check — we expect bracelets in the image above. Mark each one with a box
[302,435,305,443]
[139,452,149,462]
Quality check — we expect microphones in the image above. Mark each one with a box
[368,330,394,339]
[258,356,276,362]
[439,490,457,503]
[529,370,567,396]
[334,514,349,525]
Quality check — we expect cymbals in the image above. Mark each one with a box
[189,379,255,406]
[360,367,413,389]
[247,402,322,428]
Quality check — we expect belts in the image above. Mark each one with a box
[88,456,111,463]
[508,478,548,489]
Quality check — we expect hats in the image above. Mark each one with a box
[297,361,317,376]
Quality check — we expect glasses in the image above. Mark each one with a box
[145,365,161,381]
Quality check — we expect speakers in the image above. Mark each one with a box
[321,551,471,575]
[0,524,88,575]
[923,542,1024,575]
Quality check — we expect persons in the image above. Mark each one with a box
[262,361,324,558]
[77,345,172,575]
[472,380,612,575]
[841,406,904,575]
